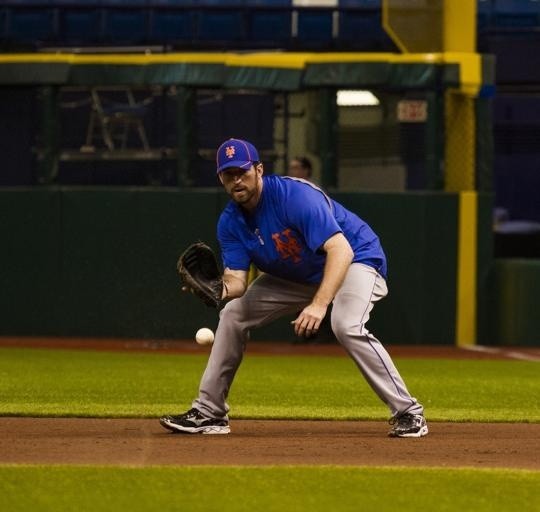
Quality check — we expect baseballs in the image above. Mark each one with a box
[195,327,214,345]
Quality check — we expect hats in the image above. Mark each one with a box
[216,137,259,173]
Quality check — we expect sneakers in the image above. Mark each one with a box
[388,414,428,438]
[160,408,231,435]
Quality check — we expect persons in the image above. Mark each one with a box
[287,155,314,181]
[157,136,428,440]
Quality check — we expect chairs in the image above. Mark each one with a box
[58,86,155,154]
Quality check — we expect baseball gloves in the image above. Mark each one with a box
[178,242,224,310]
